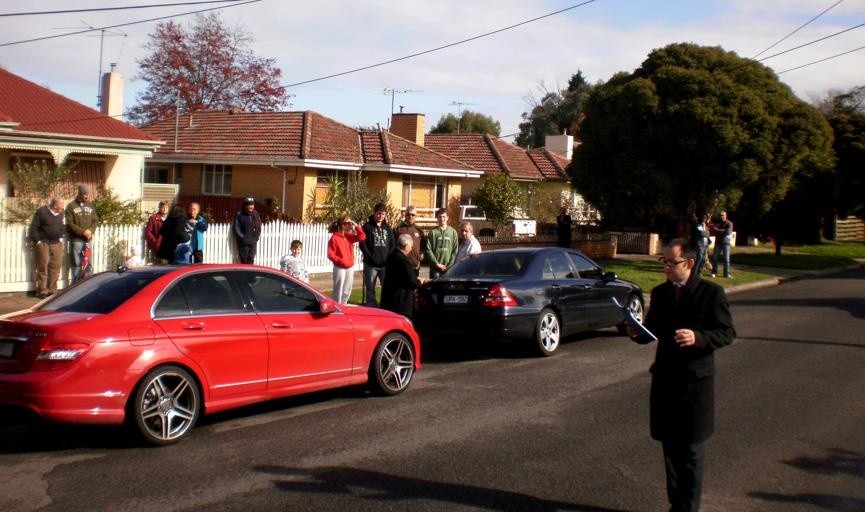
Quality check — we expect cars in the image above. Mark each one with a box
[0,262,422,448]
[411,244,648,356]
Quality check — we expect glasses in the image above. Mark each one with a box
[664,259,688,268]
[405,214,415,216]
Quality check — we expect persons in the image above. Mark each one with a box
[233,195,262,286]
[556,207,572,248]
[327,203,481,313]
[710,210,734,279]
[28,196,64,297]
[279,240,309,293]
[146,196,208,264]
[690,210,712,278]
[65,184,99,285]
[624,237,737,511]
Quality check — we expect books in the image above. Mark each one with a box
[612,297,659,344]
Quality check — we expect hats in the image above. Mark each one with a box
[243,197,254,204]
[78,185,91,193]
